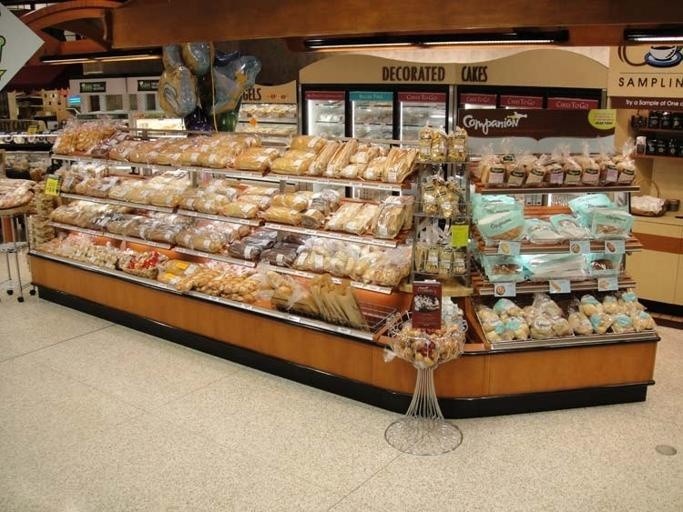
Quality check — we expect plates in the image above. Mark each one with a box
[644,52,682,68]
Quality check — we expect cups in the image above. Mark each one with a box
[650,44,683,61]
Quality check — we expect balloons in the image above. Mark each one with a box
[155,41,263,138]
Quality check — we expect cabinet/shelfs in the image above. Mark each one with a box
[5,88,64,124]
[29,131,660,420]
[608,91,682,318]
[65,76,298,133]
[300,84,448,151]
[453,82,608,116]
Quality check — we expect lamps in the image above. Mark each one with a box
[304,28,570,51]
[40,49,161,63]
[622,26,683,45]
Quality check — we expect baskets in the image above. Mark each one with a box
[629,178,667,217]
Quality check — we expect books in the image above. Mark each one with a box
[410,280,442,333]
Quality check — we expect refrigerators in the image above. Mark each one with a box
[299,82,454,199]
[453,81,607,199]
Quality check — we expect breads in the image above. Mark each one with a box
[44,125,654,343]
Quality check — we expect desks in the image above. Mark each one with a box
[0,177,37,306]
[377,305,468,456]
